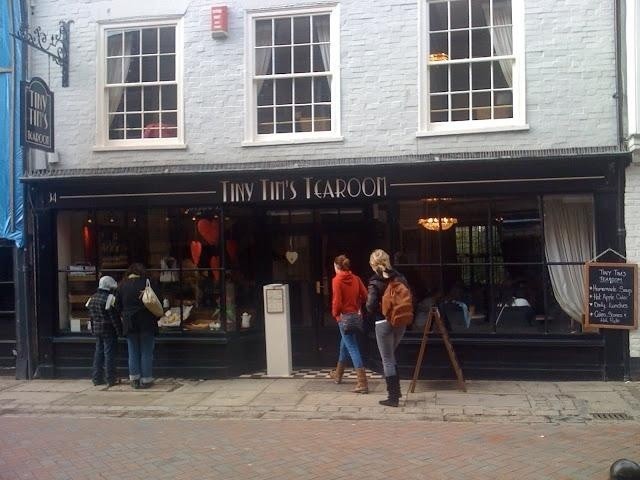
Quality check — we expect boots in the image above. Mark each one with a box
[352,368,369,394]
[380,365,402,406]
[330,363,344,384]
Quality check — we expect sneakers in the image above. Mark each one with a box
[132,379,150,389]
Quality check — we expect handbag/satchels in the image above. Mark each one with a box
[142,279,164,318]
[340,312,365,335]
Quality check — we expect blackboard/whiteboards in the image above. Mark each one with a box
[583,262,639,330]
[434,307,468,393]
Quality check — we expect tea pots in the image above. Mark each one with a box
[241,312,252,329]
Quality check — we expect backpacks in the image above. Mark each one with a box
[381,278,414,327]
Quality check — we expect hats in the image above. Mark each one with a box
[98,276,118,291]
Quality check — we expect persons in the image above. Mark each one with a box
[84,276,120,386]
[113,262,164,389]
[365,248,410,408]
[327,254,369,394]
